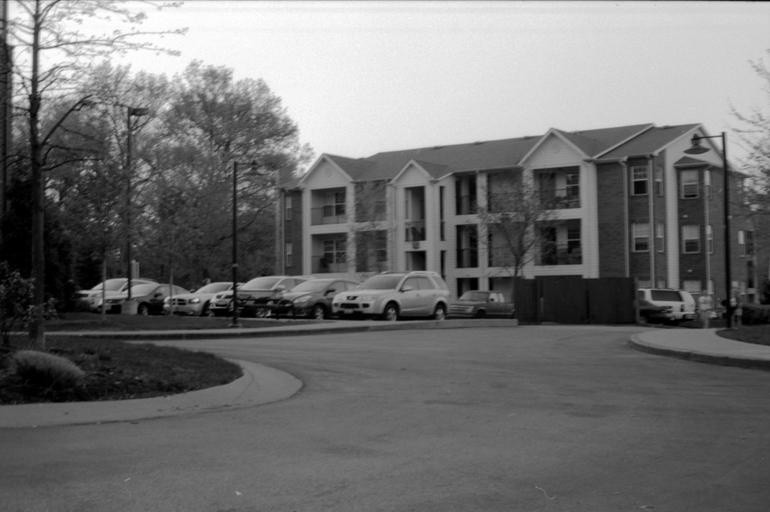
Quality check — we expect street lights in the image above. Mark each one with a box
[126,105,149,299]
[683,131,731,329]
[229,161,258,328]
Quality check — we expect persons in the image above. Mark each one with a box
[698,289,713,327]
[730,289,744,328]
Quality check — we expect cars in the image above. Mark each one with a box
[331,270,450,321]
[638,287,686,326]
[162,281,245,317]
[209,289,239,317]
[238,276,307,317]
[76,277,160,313]
[265,278,360,320]
[97,283,192,316]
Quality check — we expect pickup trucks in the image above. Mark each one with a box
[448,290,516,319]
